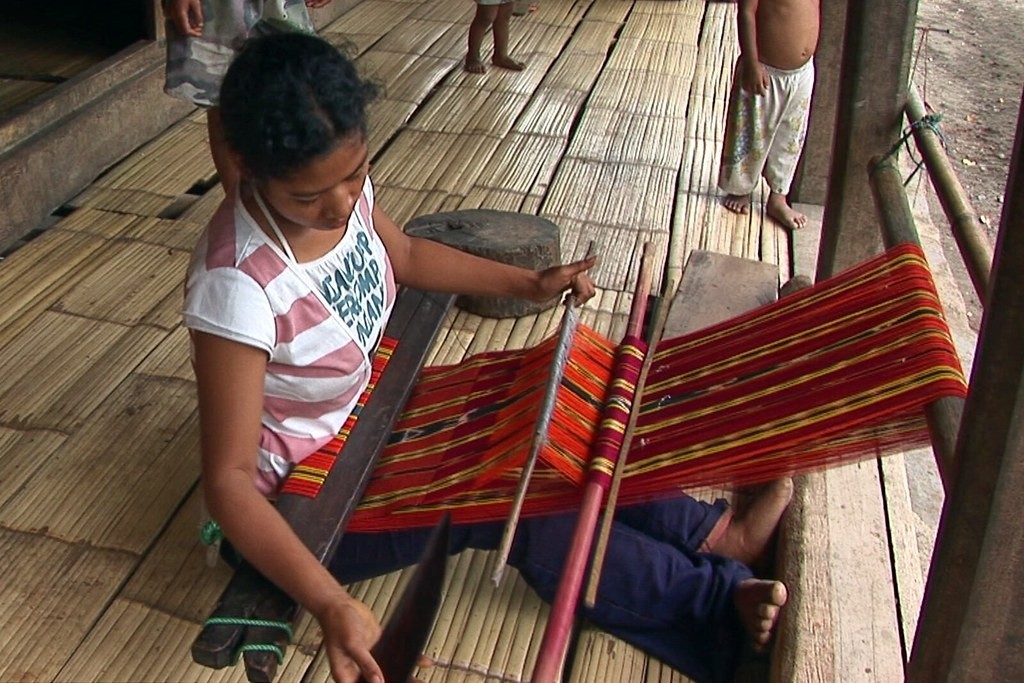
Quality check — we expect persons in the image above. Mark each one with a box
[177,18,794,683]
[466,0,537,74]
[718,0,820,230]
[161,0,319,195]
